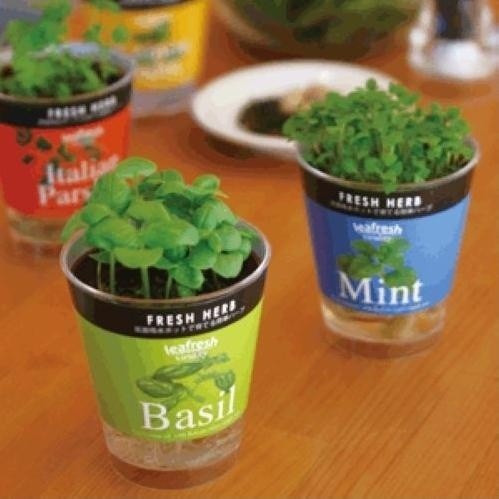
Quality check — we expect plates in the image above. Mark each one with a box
[190,60,403,156]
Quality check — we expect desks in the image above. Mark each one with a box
[2,2,497,498]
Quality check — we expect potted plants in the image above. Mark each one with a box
[217,1,417,62]
[281,77,480,346]
[1,1,141,260]
[57,155,272,472]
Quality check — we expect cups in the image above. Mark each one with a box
[292,132,477,358]
[0,44,134,252]
[54,221,277,478]
